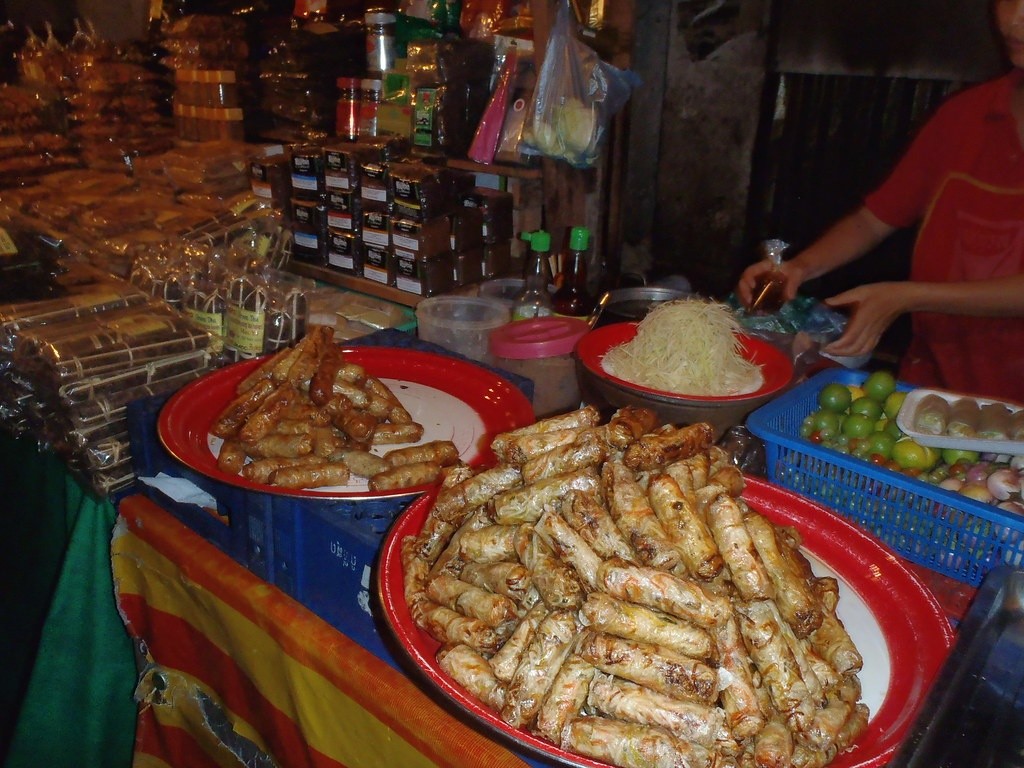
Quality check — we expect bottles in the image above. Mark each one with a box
[512,230,552,321]
[553,227,597,322]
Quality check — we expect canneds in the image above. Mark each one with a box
[335,7,398,144]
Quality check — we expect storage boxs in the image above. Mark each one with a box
[124,327,1011,767]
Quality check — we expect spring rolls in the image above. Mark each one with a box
[211,324,458,493]
[912,394,1024,444]
[402,404,871,768]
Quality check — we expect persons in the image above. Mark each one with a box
[738,0,1024,410]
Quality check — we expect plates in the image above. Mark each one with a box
[157,345,536,499]
[377,471,954,768]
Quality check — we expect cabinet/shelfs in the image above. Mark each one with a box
[248,127,568,309]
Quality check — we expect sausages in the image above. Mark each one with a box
[310,349,337,406]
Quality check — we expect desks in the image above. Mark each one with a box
[109,490,1009,768]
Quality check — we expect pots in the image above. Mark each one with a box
[602,270,699,324]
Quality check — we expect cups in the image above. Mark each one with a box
[719,425,764,474]
[365,8,396,72]
[361,79,381,142]
[335,78,360,141]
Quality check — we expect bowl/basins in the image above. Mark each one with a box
[577,322,794,445]
[477,279,554,309]
[417,296,508,360]
